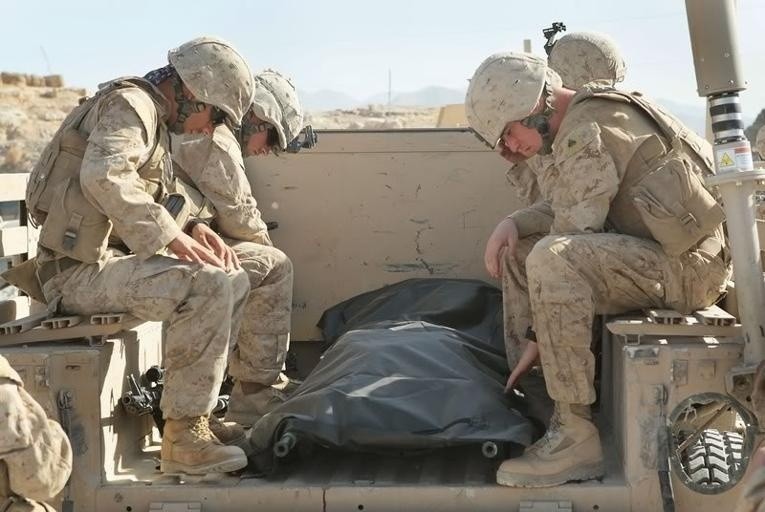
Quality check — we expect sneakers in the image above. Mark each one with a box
[493,402,608,489]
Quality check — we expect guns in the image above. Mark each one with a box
[121,364,165,436]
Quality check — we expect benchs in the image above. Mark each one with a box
[2,309,169,488]
[594,296,763,488]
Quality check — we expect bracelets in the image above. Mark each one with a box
[524,327,536,343]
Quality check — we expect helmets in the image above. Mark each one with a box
[547,32,630,96]
[252,66,306,149]
[459,50,549,148]
[166,34,248,132]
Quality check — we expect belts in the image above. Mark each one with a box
[34,252,86,287]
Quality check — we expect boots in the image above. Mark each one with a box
[157,413,249,479]
[223,377,303,428]
[689,234,734,266]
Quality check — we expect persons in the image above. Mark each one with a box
[464,49,733,489]
[0,356,73,512]
[170,68,319,427]
[500,23,627,206]
[36,37,254,475]
